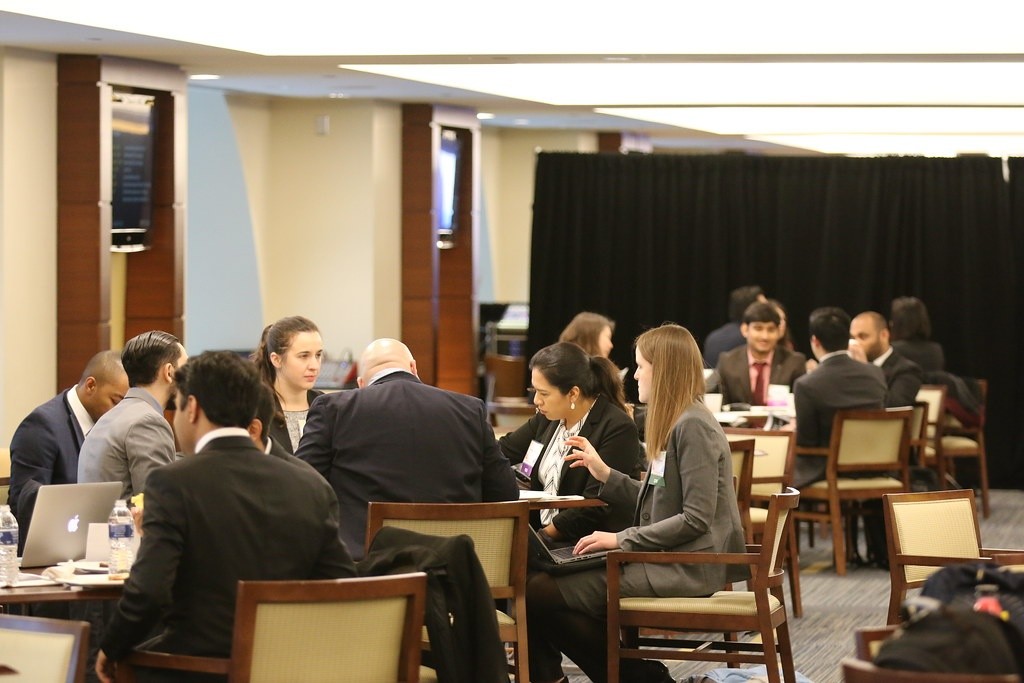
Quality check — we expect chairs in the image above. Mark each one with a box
[0,379,1024,683]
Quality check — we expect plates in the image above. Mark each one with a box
[56,561,111,573]
[55,574,125,589]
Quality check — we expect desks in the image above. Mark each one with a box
[518,494,610,507]
[0,555,126,632]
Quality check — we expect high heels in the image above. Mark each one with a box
[642,660,676,683]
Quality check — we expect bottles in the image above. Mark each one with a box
[108,501,135,578]
[0,504,19,587]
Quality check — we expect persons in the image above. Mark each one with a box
[251,316,325,456]
[497,342,641,683]
[295,339,520,562]
[526,326,753,683]
[96,351,358,683]
[703,288,946,568]
[7,352,129,558]
[561,313,613,357]
[249,384,339,523]
[77,331,188,517]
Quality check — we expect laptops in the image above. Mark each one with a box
[528,524,622,563]
[16,479,125,567]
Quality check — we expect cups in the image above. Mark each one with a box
[86,523,108,561]
[703,393,723,418]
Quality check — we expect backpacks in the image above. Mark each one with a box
[873,556,1023,675]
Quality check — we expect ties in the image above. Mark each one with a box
[754,363,765,405]
